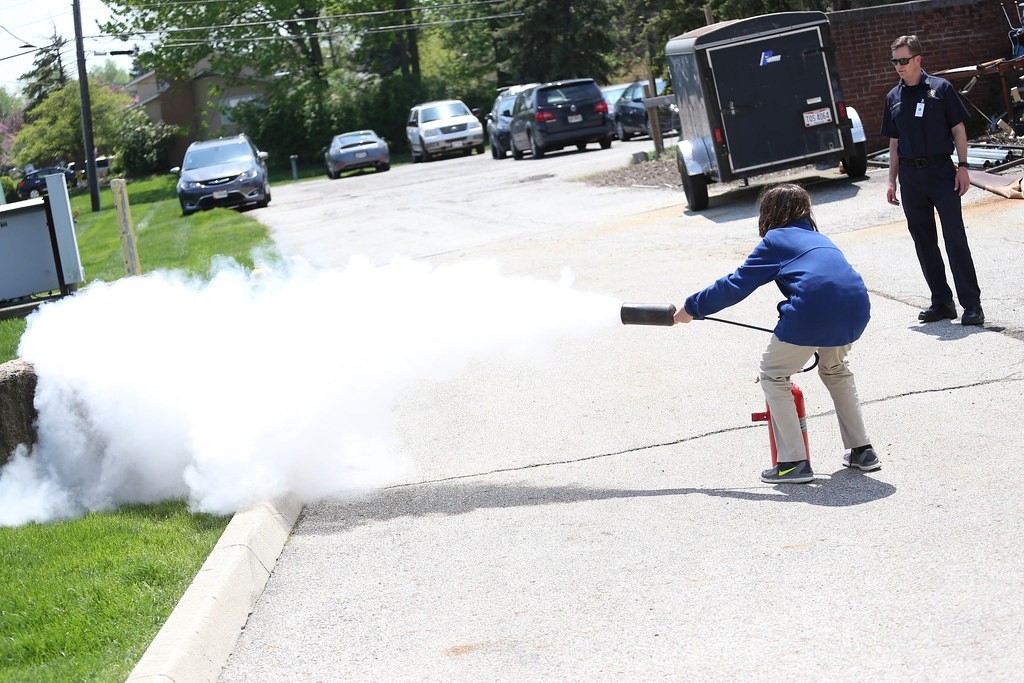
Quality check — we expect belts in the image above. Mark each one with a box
[899,156,946,169]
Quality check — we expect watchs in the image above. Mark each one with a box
[957,162,970,169]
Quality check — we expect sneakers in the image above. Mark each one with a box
[842,449,882,471]
[961,304,985,325]
[918,303,958,321]
[760,459,815,484]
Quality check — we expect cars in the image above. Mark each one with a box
[598,83,633,140]
[320,128,391,178]
[482,83,543,159]
[16,166,85,200]
[170,131,273,217]
[613,78,681,143]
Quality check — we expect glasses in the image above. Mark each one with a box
[889,53,921,66]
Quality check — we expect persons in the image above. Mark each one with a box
[672,183,881,485]
[885,35,985,327]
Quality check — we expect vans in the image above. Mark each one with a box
[82,156,114,180]
[503,78,615,161]
[405,99,485,164]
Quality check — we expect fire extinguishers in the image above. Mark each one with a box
[620,305,820,475]
